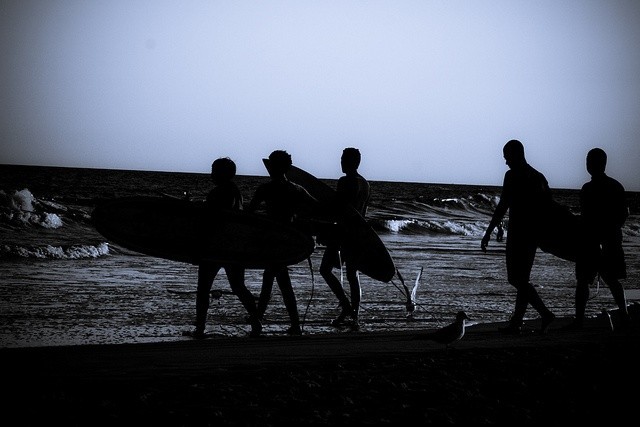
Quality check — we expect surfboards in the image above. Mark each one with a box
[529,201,627,279]
[261,156,396,284]
[91,195,316,270]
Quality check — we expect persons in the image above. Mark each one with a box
[183,156,263,340]
[247,150,315,336]
[481,139,560,321]
[320,148,371,324]
[574,149,631,317]
